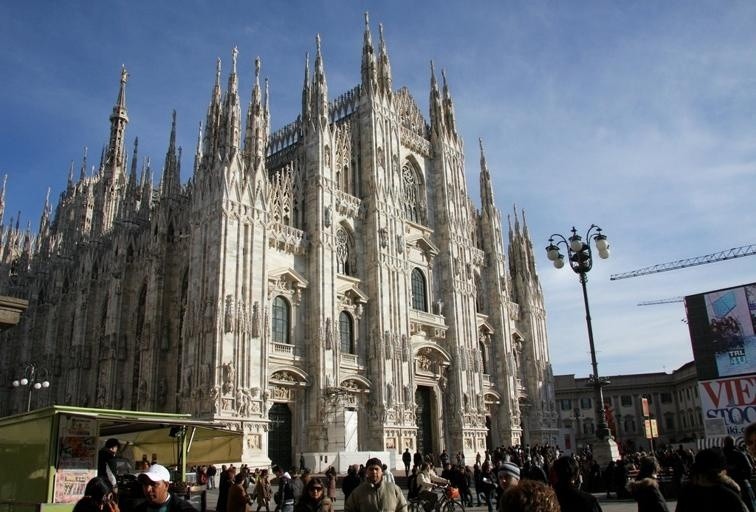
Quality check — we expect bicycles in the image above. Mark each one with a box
[407,478,465,512]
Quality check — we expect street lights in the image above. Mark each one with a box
[12,360,53,412]
[568,408,585,448]
[545,221,621,474]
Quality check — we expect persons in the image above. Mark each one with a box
[711,316,741,342]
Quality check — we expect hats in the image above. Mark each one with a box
[498,461,520,480]
[138,464,170,482]
[365,457,382,468]
